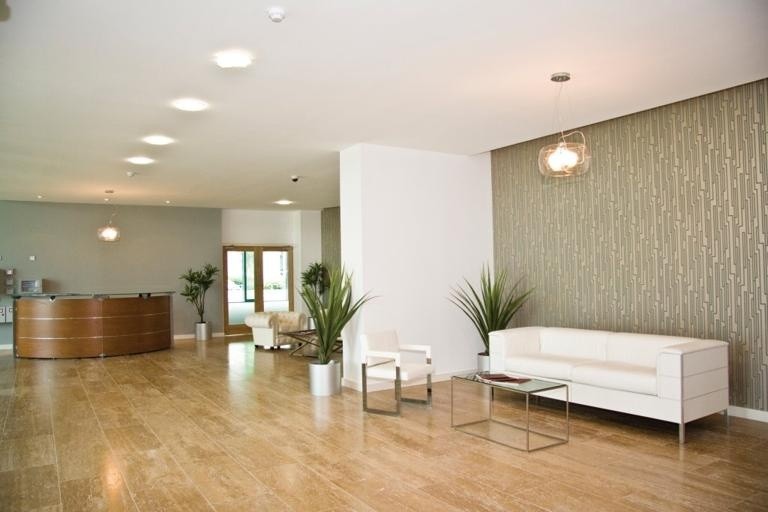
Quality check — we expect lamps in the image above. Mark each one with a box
[97,189,122,241]
[537,73,592,178]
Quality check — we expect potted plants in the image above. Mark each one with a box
[298,263,382,398]
[446,263,536,373]
[178,264,221,340]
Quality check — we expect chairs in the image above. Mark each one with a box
[360,329,435,415]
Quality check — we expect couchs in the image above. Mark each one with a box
[489,325,730,445]
[245,311,307,350]
